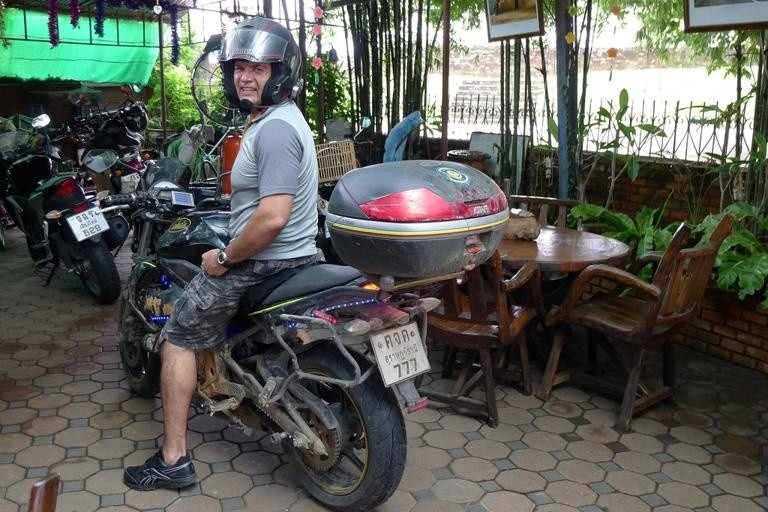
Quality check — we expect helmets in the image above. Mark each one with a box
[123,99,149,134]
[217,17,303,111]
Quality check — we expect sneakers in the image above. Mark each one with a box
[125,448,198,491]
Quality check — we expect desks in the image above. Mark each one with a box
[442,223,635,391]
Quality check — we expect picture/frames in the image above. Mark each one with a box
[683,0,768,33]
[484,0,545,42]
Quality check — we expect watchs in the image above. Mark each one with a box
[216,247,232,270]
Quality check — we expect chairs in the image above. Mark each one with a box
[503,179,585,226]
[535,213,735,432]
[414,248,546,428]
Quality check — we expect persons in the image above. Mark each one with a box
[122,17,320,492]
